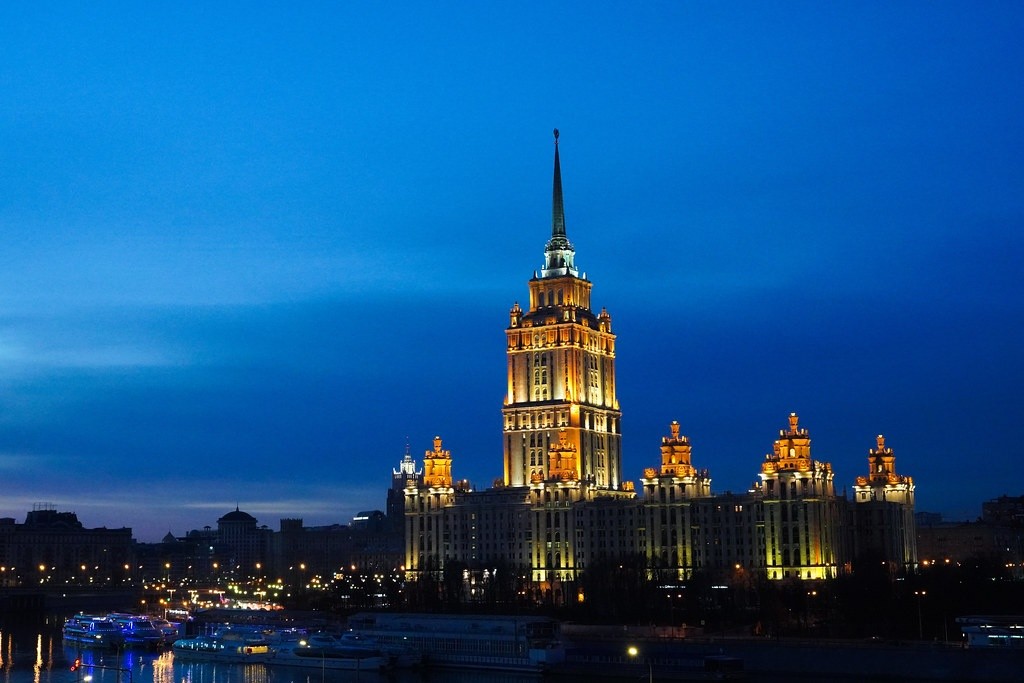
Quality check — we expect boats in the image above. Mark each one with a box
[305,635,422,667]
[62,610,182,652]
[340,612,577,656]
[172,624,390,670]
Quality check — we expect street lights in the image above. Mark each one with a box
[40,564,55,584]
[915,592,926,639]
[166,563,170,586]
[667,594,682,625]
[159,599,168,618]
[213,563,219,584]
[807,591,817,638]
[142,599,148,616]
[626,645,653,683]
[1,567,15,586]
